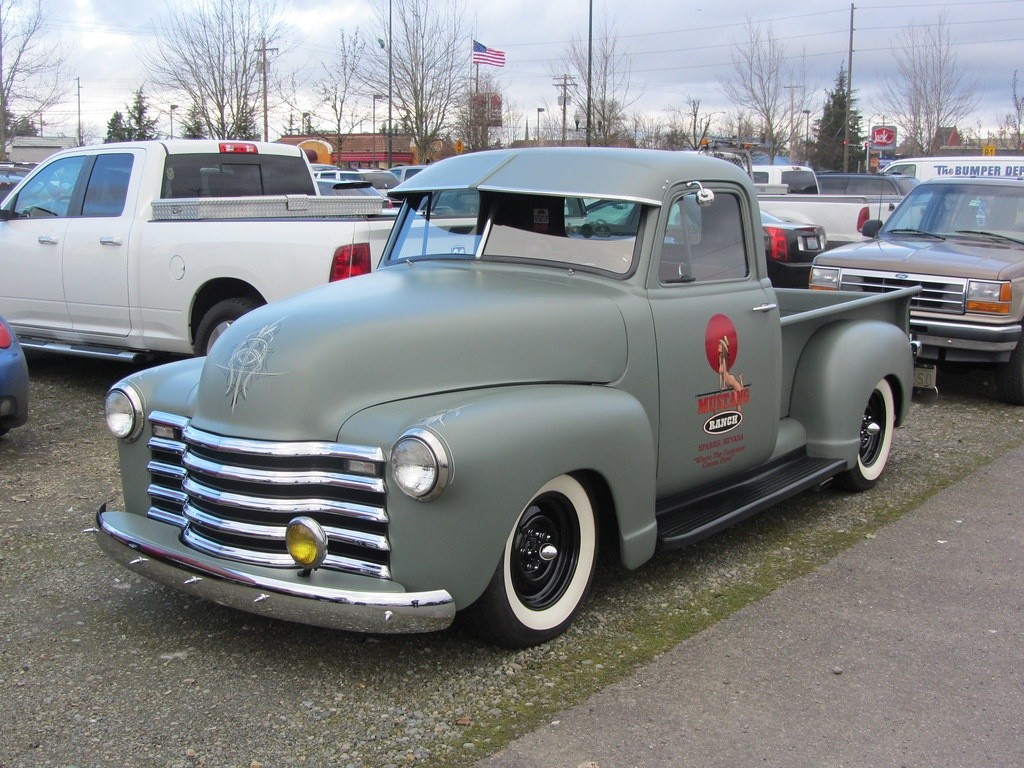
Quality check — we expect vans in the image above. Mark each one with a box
[873,156,1024,204]
[746,164,820,197]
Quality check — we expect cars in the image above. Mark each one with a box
[561,200,828,293]
[806,174,1024,409]
[305,160,483,216]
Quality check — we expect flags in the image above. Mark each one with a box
[473,40,505,67]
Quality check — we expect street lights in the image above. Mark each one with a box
[170,104,179,139]
[803,110,810,160]
[372,94,383,170]
[536,108,546,147]
[699,111,728,139]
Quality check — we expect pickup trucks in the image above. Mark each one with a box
[1,148,480,365]
[92,148,923,652]
[674,147,934,252]
